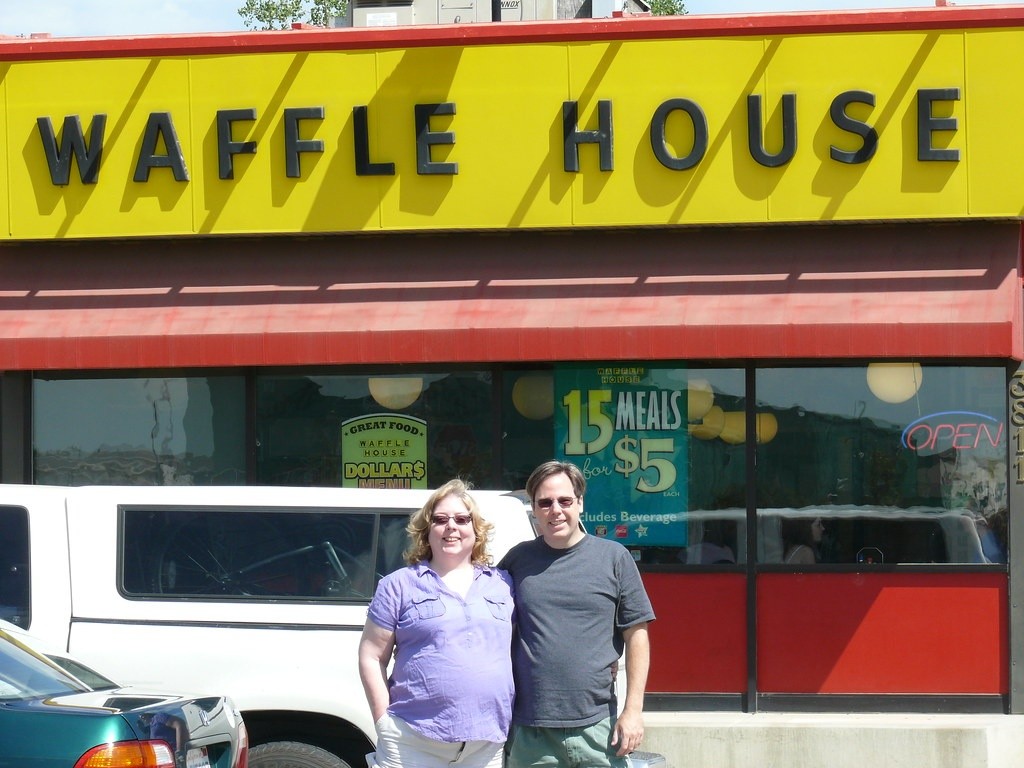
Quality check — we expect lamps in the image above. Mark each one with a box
[513,374,556,421]
[866,361,923,405]
[693,405,725,440]
[719,412,747,444]
[688,380,714,422]
[756,413,778,445]
[368,377,423,409]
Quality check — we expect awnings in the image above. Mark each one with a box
[1,220,1024,373]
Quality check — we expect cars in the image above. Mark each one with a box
[1,619,250,768]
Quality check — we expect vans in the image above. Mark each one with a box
[1,483,651,767]
[600,504,1005,568]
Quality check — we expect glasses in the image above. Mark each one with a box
[430,515,473,524]
[533,496,580,508]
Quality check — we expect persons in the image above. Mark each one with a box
[357,478,620,767]
[495,460,656,768]
[607,443,1000,565]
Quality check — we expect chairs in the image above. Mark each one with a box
[848,538,887,565]
